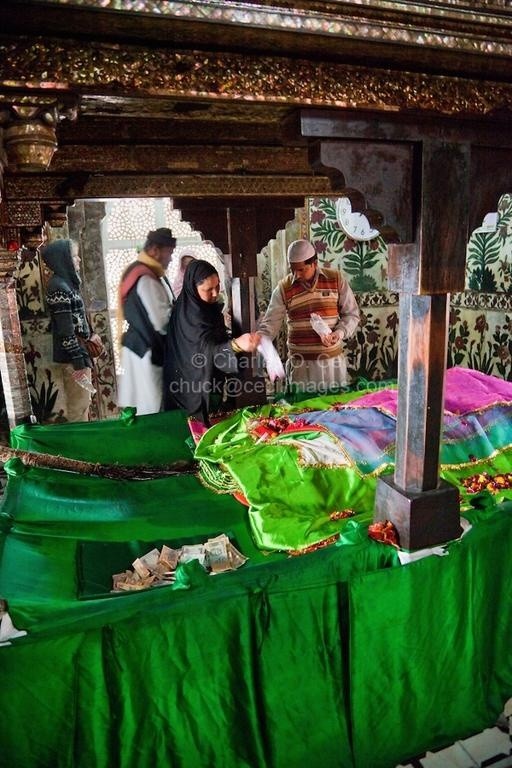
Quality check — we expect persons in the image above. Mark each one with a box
[114,226,177,416]
[249,239,361,394]
[162,259,261,429]
[172,248,199,302]
[40,237,104,423]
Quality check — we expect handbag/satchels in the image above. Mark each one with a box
[78,332,105,359]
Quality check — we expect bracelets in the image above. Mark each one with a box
[230,337,244,354]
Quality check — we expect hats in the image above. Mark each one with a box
[147,227,176,248]
[287,240,316,264]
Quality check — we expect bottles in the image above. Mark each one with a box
[69,366,96,395]
[310,312,332,338]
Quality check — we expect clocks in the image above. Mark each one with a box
[336,197,380,242]
